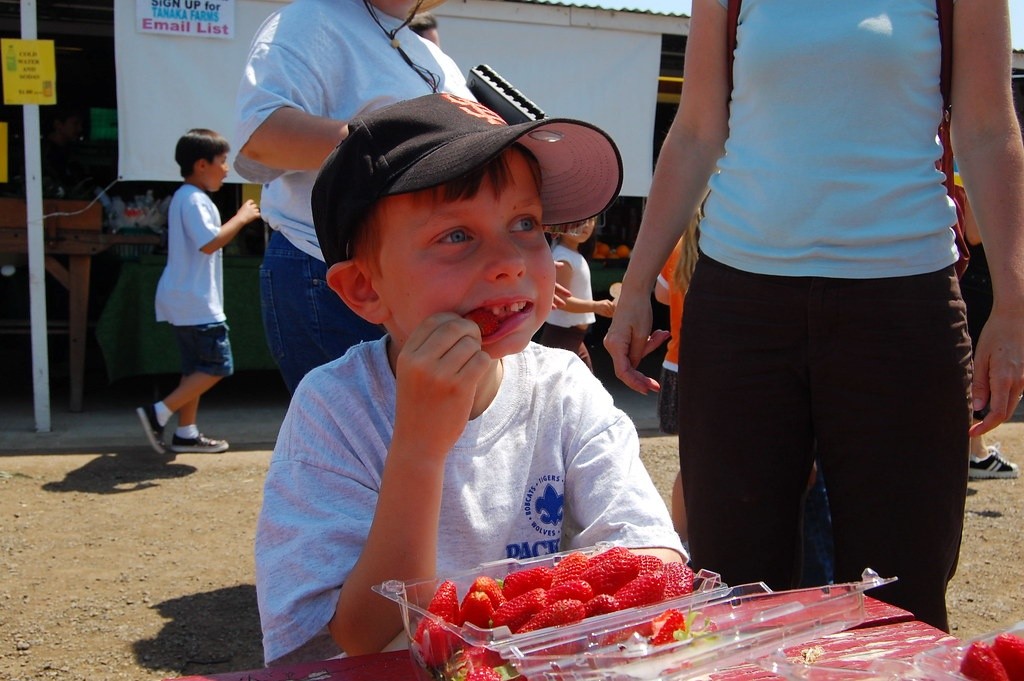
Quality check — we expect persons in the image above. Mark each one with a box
[532,213,617,378]
[133,129,263,458]
[932,130,1024,479]
[252,93,691,670]
[233,0,508,401]
[672,469,691,554]
[40,105,82,175]
[602,0,1024,634]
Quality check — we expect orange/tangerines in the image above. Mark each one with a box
[593,241,632,259]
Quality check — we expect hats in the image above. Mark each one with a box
[312,94,624,270]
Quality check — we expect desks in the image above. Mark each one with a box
[92,254,627,402]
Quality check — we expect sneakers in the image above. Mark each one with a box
[171,434,229,453]
[968,443,1019,478]
[137,405,165,454]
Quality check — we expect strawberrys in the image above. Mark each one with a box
[413,546,695,681]
[959,632,1024,681]
[464,308,500,338]
